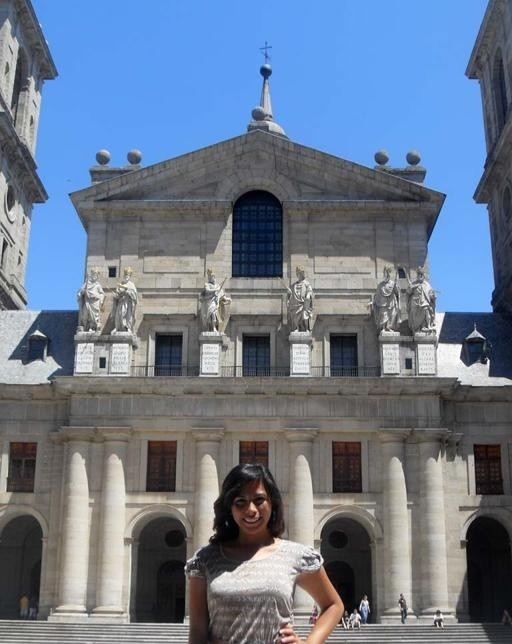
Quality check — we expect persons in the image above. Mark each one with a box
[309,594,371,630]
[433,610,445,630]
[406,265,437,334]
[501,608,512,625]
[76,268,106,332]
[285,266,315,333]
[19,592,29,620]
[201,267,232,332]
[398,594,409,624]
[374,265,401,331]
[184,463,345,644]
[28,593,37,620]
[112,266,138,333]
